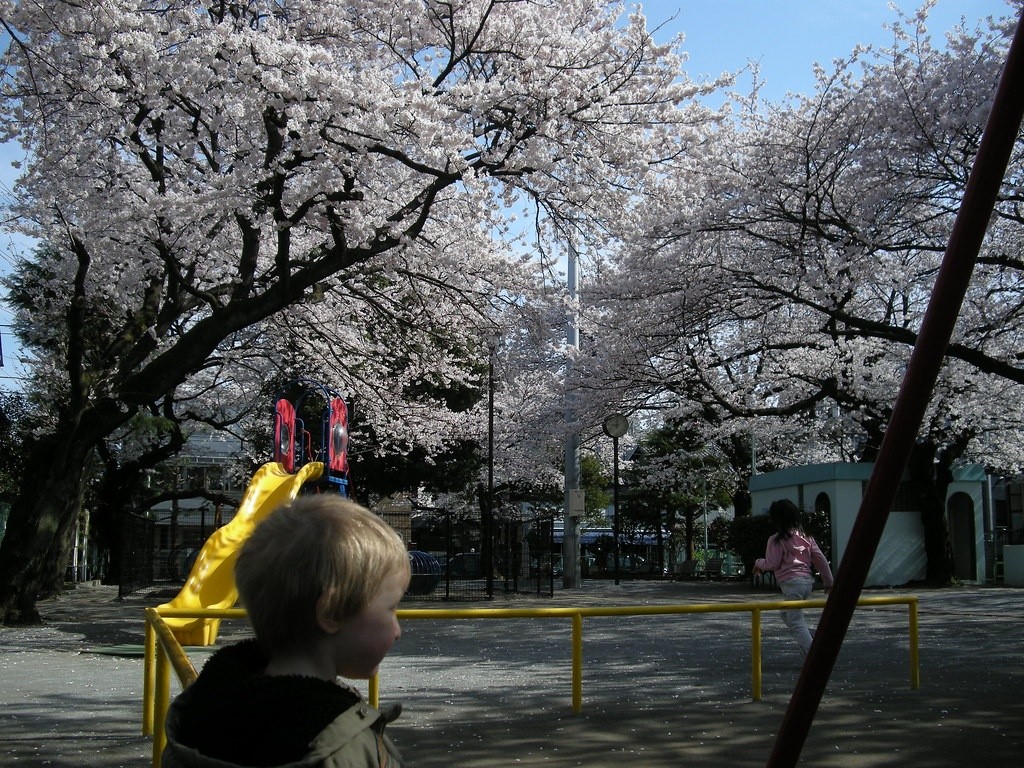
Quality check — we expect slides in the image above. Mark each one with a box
[146,460,324,646]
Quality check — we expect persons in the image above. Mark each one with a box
[159,495,412,768]
[752,499,835,659]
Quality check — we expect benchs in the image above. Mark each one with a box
[667,559,777,587]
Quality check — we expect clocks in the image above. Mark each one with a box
[602,413,629,438]
